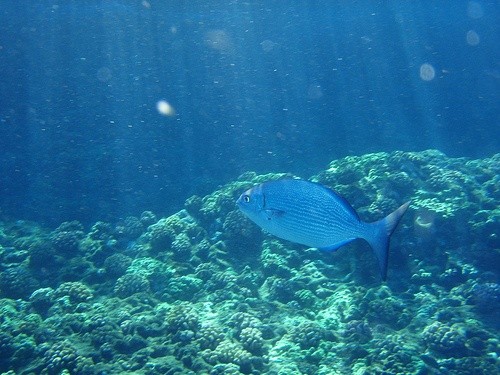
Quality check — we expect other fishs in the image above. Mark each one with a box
[236,178,412,282]
